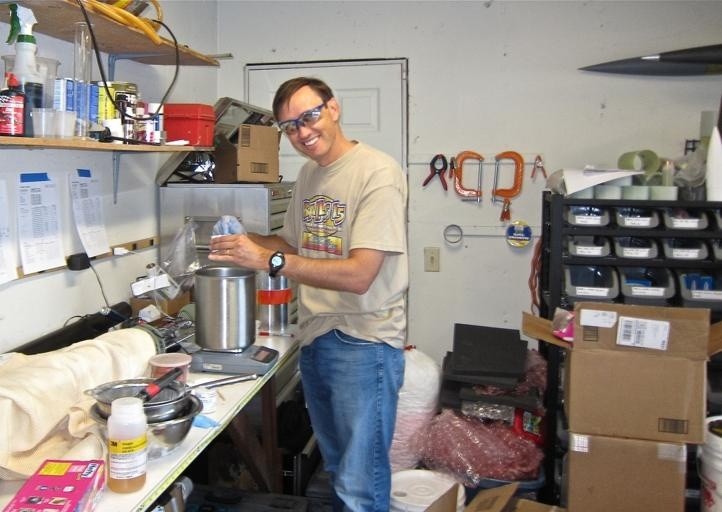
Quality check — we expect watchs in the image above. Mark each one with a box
[269,251,284,279]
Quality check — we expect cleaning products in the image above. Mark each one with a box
[6,4,43,136]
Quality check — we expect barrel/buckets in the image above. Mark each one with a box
[389,470,467,512]
[697,415,722,512]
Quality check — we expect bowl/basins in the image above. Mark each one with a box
[32,108,77,138]
[89,395,202,458]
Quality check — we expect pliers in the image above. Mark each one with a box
[422,154,447,191]
[531,155,546,178]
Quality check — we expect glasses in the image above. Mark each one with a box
[277,103,324,135]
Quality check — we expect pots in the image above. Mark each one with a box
[91,372,258,426]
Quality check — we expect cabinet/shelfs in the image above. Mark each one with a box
[0,0,222,154]
[539,191,721,511]
[144,376,300,512]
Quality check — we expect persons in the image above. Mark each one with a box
[208,77,408,512]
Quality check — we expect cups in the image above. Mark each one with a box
[67,254,91,269]
[2,56,61,109]
[150,352,193,384]
[71,20,95,142]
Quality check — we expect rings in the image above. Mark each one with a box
[226,249,230,255]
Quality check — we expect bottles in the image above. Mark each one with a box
[0,73,26,137]
[108,395,148,494]
[662,160,675,188]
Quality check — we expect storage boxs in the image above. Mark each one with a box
[214,125,285,183]
[418,478,563,511]
[566,430,689,512]
[520,301,721,444]
